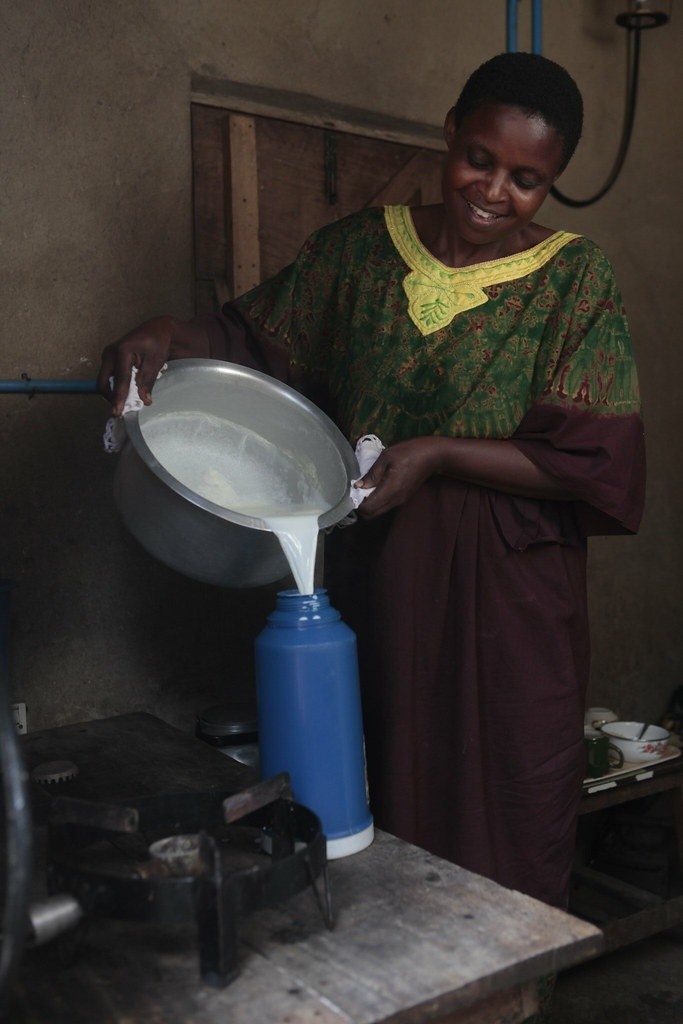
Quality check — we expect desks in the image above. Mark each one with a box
[1,712,608,1024]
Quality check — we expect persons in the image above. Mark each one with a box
[96,52,649,913]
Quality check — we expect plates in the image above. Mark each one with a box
[582,745,682,783]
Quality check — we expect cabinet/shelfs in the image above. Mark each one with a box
[573,767,682,950]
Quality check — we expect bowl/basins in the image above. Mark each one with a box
[585,706,619,729]
[599,721,671,763]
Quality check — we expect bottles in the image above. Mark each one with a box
[254,589,374,859]
[197,701,259,771]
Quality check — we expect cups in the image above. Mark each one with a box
[582,734,624,779]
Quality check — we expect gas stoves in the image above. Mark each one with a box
[16,771,333,987]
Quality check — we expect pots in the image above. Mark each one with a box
[112,359,358,587]
